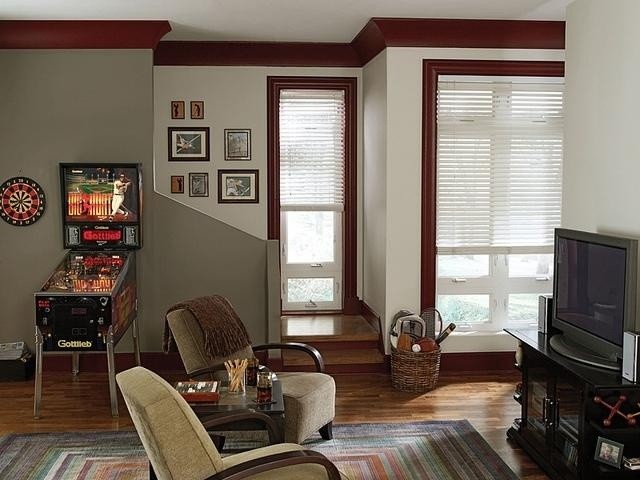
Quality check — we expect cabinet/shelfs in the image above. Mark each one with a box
[503,326,640,480]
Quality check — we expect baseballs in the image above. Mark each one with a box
[412,344,421,352]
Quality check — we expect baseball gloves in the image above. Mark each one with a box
[398,334,418,351]
[416,336,438,352]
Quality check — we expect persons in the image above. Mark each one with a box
[600,445,615,464]
[109,172,132,222]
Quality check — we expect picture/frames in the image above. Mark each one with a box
[171,175,184,192]
[223,129,251,160]
[594,437,624,469]
[171,101,185,119]
[217,169,259,203]
[189,173,209,197]
[168,126,210,161]
[190,100,204,119]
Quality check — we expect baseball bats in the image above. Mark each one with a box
[435,323,455,344]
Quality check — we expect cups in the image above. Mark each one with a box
[245,359,259,386]
[257,371,273,404]
[227,366,247,395]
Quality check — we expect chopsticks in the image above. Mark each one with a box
[224,358,249,392]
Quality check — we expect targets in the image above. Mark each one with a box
[0,177,45,226]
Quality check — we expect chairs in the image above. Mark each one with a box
[116,366,341,480]
[160,294,336,443]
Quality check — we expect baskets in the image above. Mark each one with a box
[391,345,442,395]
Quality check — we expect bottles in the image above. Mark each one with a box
[515,340,522,365]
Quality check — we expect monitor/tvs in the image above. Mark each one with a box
[59,163,143,222]
[549,228,640,371]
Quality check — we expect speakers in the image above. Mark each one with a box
[538,295,563,334]
[622,331,640,384]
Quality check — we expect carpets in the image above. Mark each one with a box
[0,419,520,480]
